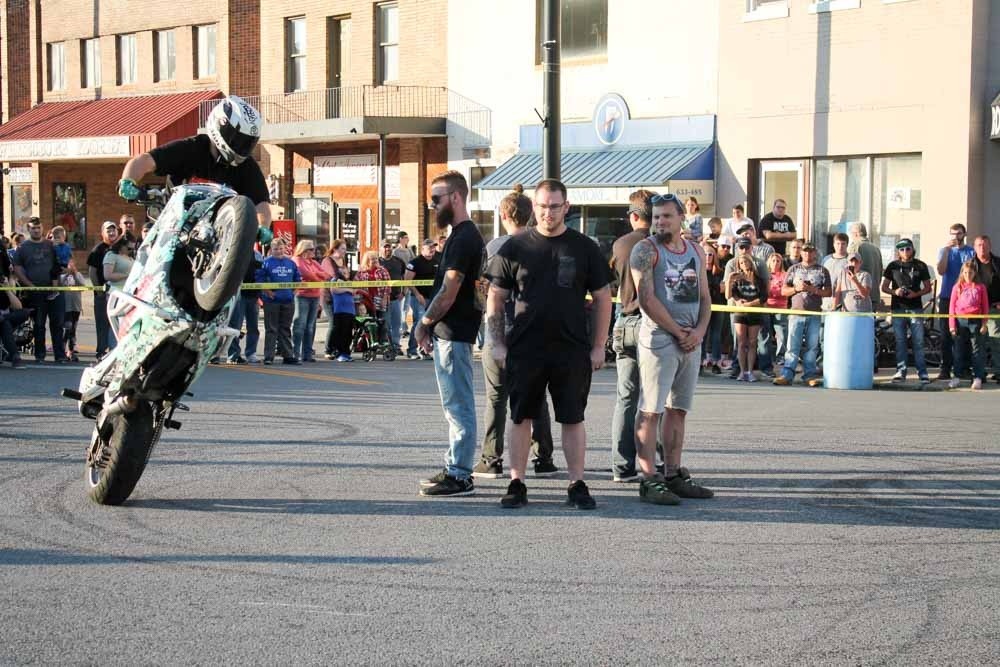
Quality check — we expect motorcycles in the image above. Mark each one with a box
[61,176,275,504]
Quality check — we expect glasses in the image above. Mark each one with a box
[650,193,685,213]
[704,252,714,258]
[431,191,454,204]
[533,203,566,213]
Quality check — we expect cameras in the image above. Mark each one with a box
[902,286,912,295]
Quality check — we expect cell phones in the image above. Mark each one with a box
[849,266,854,275]
[805,281,811,286]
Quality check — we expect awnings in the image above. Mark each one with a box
[472,143,714,210]
[0,91,222,161]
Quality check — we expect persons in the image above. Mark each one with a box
[936,223,1000,388]
[0,217,86,369]
[630,195,714,504]
[473,184,559,477]
[681,197,832,385]
[486,180,617,510]
[208,238,331,364]
[89,216,154,361]
[118,94,273,257]
[415,171,488,496]
[613,191,689,482]
[821,222,883,374]
[320,240,356,362]
[881,238,932,383]
[355,231,446,360]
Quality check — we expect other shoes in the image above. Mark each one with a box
[892,373,1000,390]
[700,358,819,387]
[48,290,60,301]
[210,353,354,365]
[613,472,640,483]
[472,458,557,478]
[13,346,104,367]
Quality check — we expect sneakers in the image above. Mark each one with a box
[636,467,715,505]
[501,478,598,509]
[419,470,476,497]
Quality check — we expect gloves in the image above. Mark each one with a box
[115,179,143,202]
[257,225,274,245]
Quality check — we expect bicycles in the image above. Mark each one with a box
[783,304,842,363]
[874,298,947,366]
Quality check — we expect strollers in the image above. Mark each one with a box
[11,281,34,355]
[350,289,396,362]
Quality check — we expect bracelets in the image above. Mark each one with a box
[794,288,798,294]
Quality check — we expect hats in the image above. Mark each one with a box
[895,238,913,248]
[847,252,862,261]
[379,238,393,247]
[100,221,117,231]
[422,238,437,246]
[719,223,753,249]
[802,243,816,251]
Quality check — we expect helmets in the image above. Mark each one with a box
[206,95,261,168]
